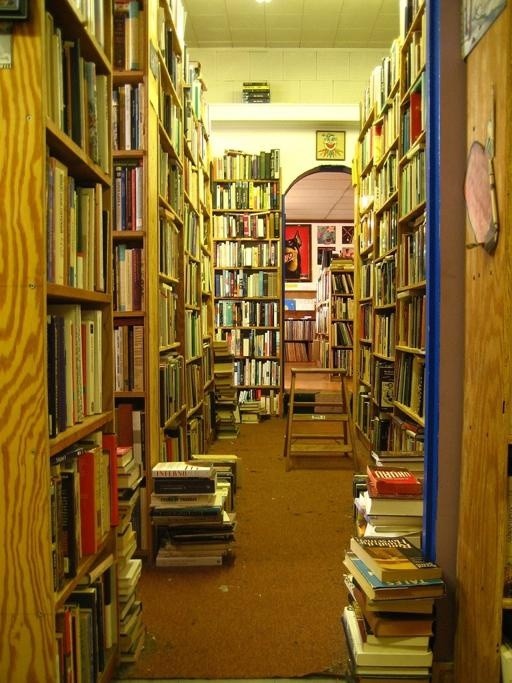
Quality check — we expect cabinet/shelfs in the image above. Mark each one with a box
[213,149,283,419]
[354,3,426,471]
[1,0,123,683]
[285,290,318,364]
[114,0,214,565]
[319,269,355,380]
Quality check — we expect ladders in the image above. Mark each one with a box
[283,367,360,472]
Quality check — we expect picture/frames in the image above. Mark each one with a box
[285,222,314,283]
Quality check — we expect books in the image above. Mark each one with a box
[150,454,240,567]
[160,1,216,461]
[48,0,115,682]
[393,0,428,452]
[357,36,399,450]
[214,148,281,438]
[316,250,354,377]
[342,451,447,683]
[109,0,146,668]
[283,321,318,362]
